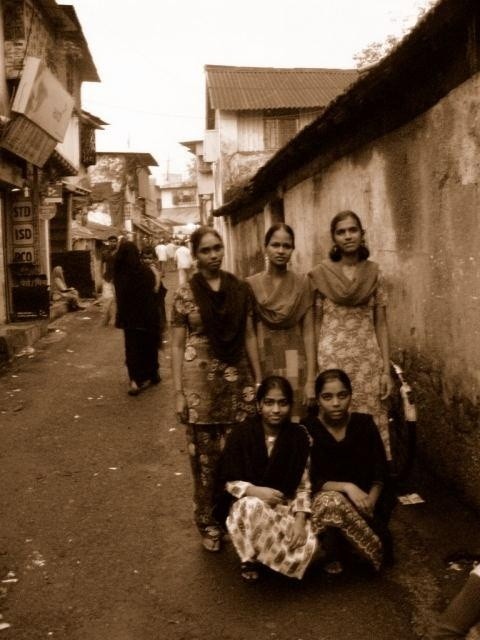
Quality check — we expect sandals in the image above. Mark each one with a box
[127,381,149,396]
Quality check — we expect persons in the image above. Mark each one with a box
[243,223,318,423]
[211,376,318,585]
[300,370,394,578]
[307,210,392,463]
[170,227,263,553]
[52,265,85,311]
[100,236,193,396]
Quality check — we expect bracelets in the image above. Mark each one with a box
[255,383,262,386]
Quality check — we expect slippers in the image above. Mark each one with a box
[202,531,223,551]
[319,550,343,574]
[241,564,274,582]
